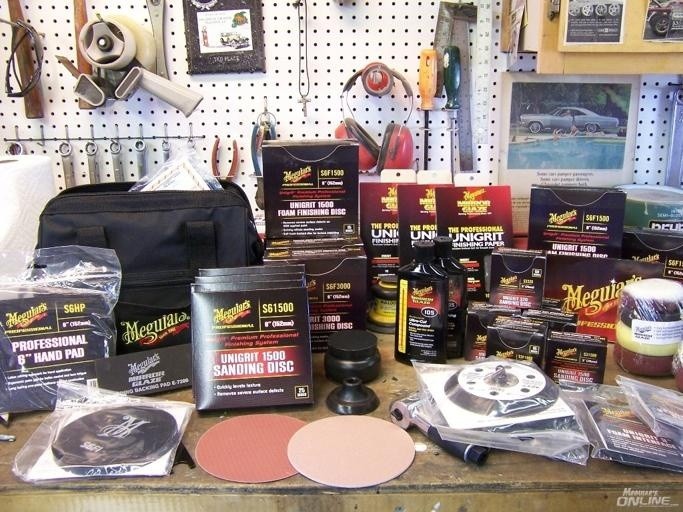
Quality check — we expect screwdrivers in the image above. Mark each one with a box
[418,46,461,184]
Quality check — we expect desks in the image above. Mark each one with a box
[0,323,683,512]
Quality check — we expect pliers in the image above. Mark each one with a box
[212,138,237,189]
[251,121,276,210]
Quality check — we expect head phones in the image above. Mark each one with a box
[340,68,414,172]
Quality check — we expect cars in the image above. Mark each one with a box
[647,0,682,37]
[519,106,620,134]
[220,31,249,49]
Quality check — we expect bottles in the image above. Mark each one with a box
[373,275,397,318]
[394,235,468,368]
